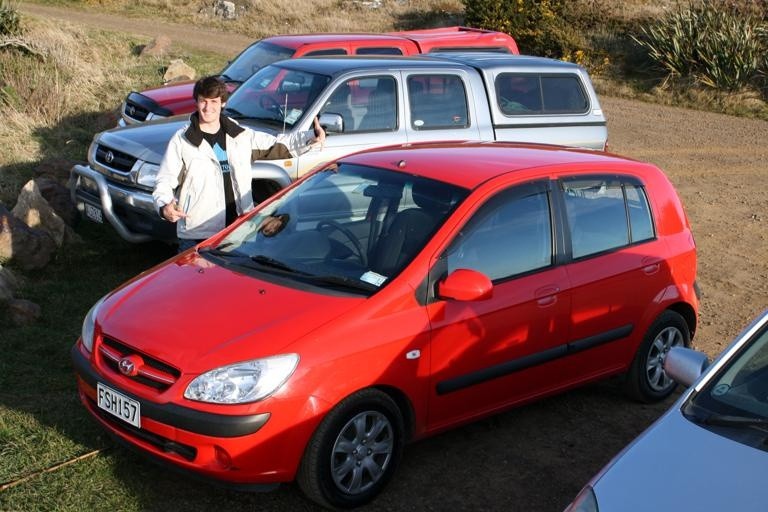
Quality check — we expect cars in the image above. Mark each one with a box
[70,139,703,512]
[561,309,768,512]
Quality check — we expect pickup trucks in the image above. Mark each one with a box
[65,50,609,262]
[112,25,521,129]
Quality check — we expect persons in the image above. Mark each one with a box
[151,76,326,253]
[174,163,338,269]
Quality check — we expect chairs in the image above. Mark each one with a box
[375,181,452,283]
[323,78,423,131]
